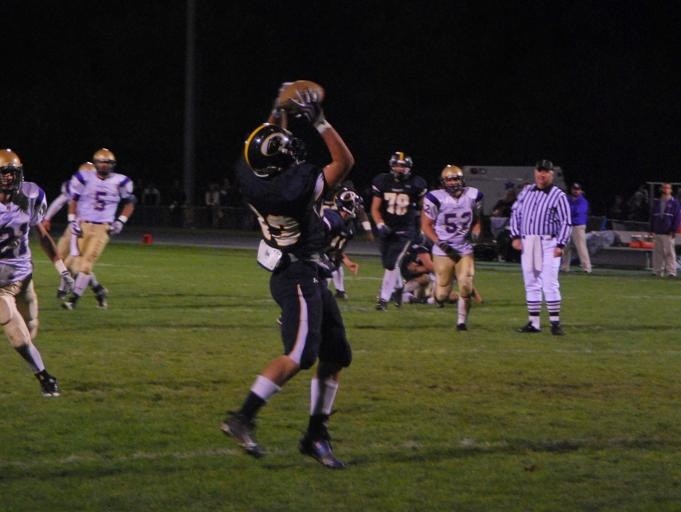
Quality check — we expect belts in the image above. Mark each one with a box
[87,220,112,225]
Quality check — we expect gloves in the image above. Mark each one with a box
[441,240,461,262]
[288,87,331,133]
[107,216,128,236]
[68,214,82,237]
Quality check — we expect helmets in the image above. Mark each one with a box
[244,123,307,178]
[0,149,24,202]
[78,148,116,176]
[333,152,465,220]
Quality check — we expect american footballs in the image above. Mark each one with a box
[276,80,323,115]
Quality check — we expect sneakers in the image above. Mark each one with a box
[41,285,108,397]
[221,410,263,457]
[517,321,540,332]
[298,433,343,468]
[335,288,467,330]
[551,322,562,335]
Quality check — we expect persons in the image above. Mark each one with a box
[137,177,237,207]
[322,187,360,299]
[650,183,681,278]
[61,148,136,311]
[219,78,355,468]
[0,148,73,396]
[559,179,591,275]
[42,161,98,298]
[370,153,484,333]
[509,159,571,335]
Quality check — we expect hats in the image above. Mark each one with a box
[536,160,552,171]
